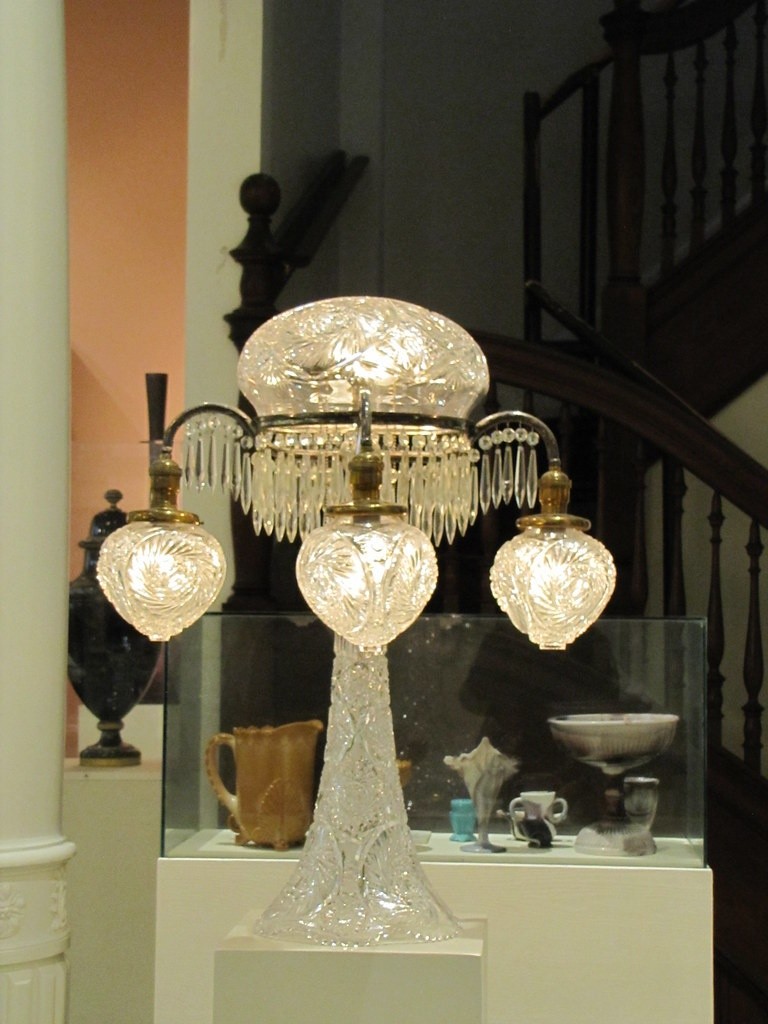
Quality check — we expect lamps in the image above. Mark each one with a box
[95,296,617,944]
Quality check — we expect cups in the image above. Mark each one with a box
[508,790,568,847]
